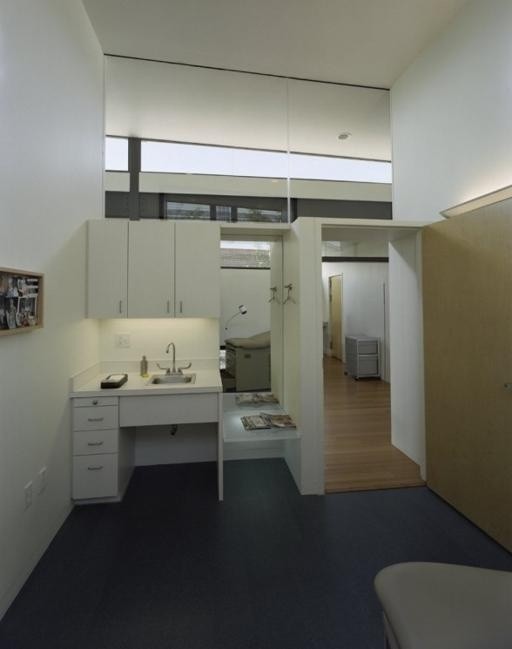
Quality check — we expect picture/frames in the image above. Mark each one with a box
[1,266,45,335]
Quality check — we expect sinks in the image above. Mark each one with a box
[144,374,196,384]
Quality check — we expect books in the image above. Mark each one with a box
[241,414,297,429]
[235,392,278,404]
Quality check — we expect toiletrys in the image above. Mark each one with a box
[140,356,147,376]
[141,356,147,376]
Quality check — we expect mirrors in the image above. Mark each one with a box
[219,232,285,394]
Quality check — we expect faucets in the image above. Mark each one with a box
[166,343,175,372]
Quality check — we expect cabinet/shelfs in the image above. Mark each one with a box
[72,397,136,505]
[128,219,223,318]
[87,219,128,318]
[348,334,381,380]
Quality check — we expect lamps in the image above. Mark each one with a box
[224,304,248,330]
